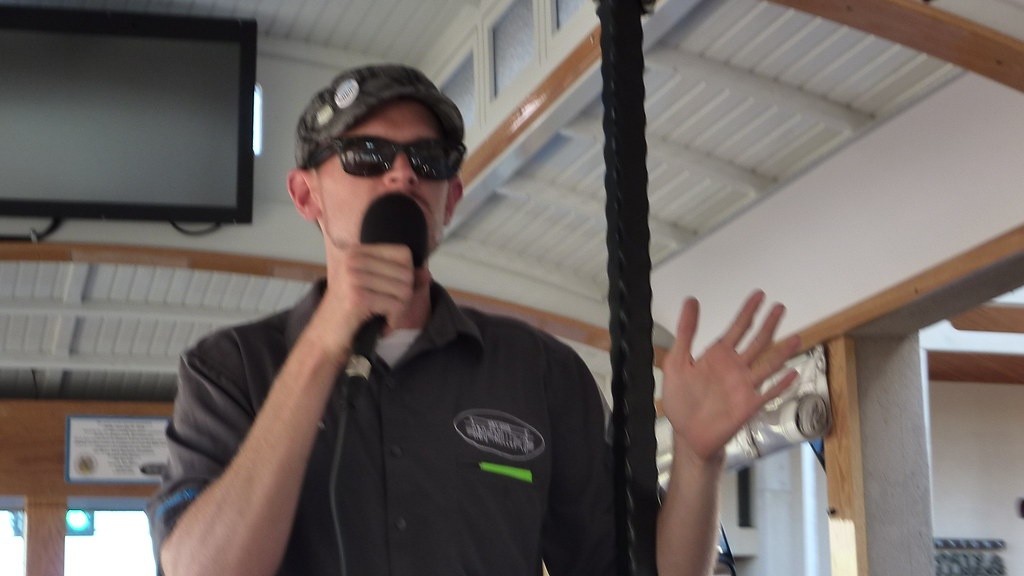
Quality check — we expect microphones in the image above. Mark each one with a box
[345,191,427,384]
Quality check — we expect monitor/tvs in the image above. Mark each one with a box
[0,7,258,225]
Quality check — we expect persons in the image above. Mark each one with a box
[147,62,799,576]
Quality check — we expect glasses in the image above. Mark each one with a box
[302,132,463,185]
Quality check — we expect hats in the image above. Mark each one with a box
[297,63,465,164]
